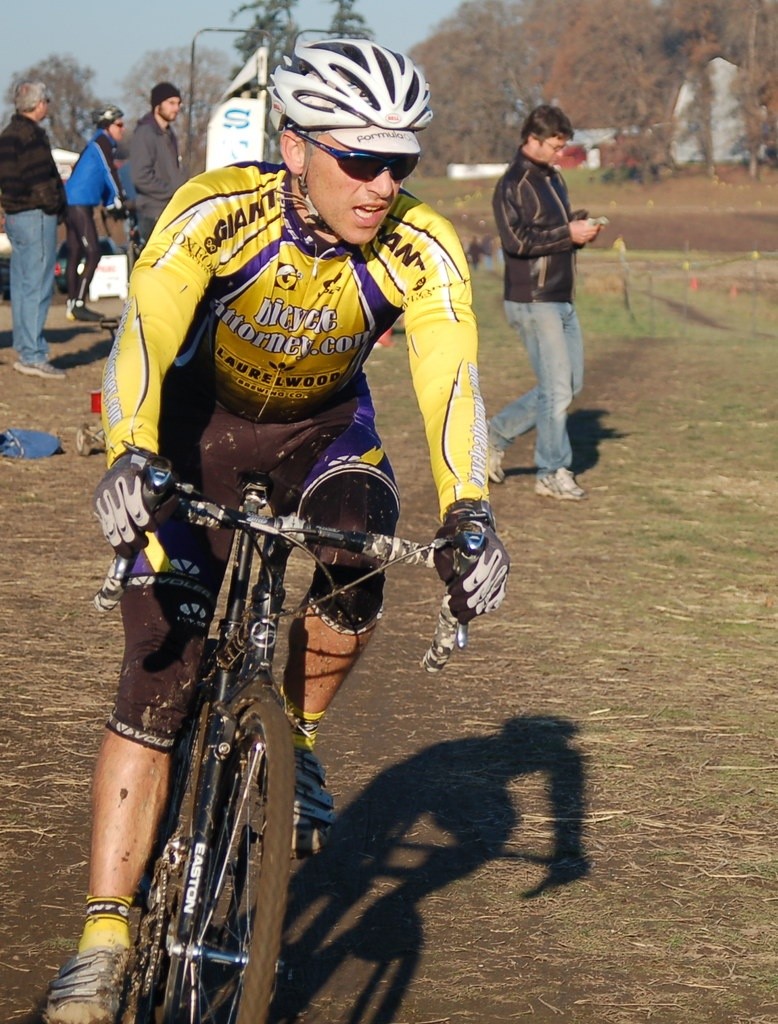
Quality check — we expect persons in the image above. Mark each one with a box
[0,81,67,381]
[127,81,186,266]
[63,104,126,319]
[37,38,509,1024]
[486,104,607,500]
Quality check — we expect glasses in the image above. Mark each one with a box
[536,134,569,156]
[111,122,124,128]
[283,125,421,184]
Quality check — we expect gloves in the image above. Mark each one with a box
[91,441,178,559]
[432,494,510,626]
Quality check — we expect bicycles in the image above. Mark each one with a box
[93,437,490,1024]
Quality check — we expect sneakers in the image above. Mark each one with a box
[41,943,130,1024]
[534,467,585,500]
[256,736,334,856]
[485,419,506,484]
[12,356,67,381]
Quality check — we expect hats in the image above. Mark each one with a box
[150,82,182,109]
[293,69,422,155]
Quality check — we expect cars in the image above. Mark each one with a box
[0,255,13,302]
[54,235,125,296]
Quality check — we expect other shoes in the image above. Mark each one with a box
[66,302,105,321]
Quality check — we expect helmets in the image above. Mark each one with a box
[266,38,435,131]
[92,105,125,128]
